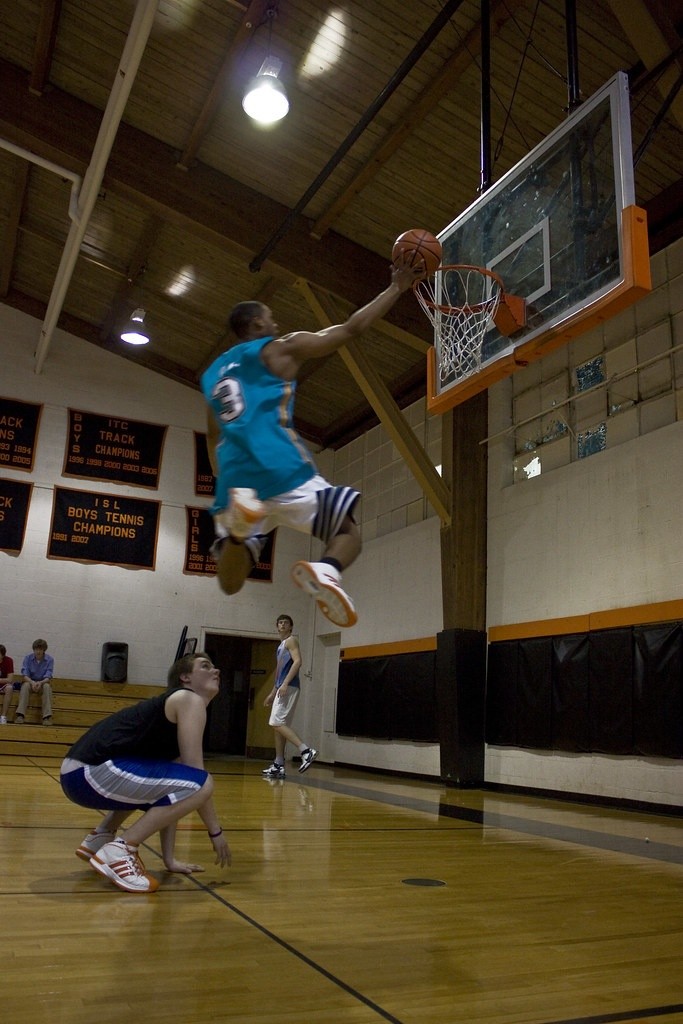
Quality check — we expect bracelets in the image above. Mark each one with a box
[209,827,223,837]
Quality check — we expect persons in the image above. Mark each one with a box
[15,639,54,725]
[0,645,14,725]
[59,653,232,892]
[263,614,318,776]
[200,248,425,628]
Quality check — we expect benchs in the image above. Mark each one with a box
[0,675,168,759]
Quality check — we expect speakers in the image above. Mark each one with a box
[101,642,129,684]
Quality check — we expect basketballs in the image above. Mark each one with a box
[390,228,444,278]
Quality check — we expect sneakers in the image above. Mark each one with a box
[229,487,269,537]
[298,748,319,773]
[14,716,23,723]
[291,560,358,627]
[89,841,159,892]
[43,717,53,725]
[75,829,117,863]
[0,716,7,724]
[262,761,286,778]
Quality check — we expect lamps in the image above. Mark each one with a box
[242,75,290,123]
[120,320,150,345]
